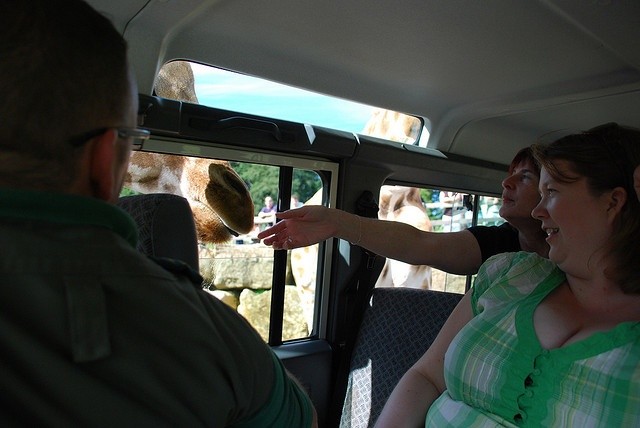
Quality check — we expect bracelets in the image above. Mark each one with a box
[350,214,362,245]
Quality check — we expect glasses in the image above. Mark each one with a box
[63,125,150,151]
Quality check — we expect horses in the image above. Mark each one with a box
[290,185,434,337]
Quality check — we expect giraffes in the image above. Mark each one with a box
[291,107,432,335]
[120,61,254,243]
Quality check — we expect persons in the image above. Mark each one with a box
[374,122,639,427]
[259,196,278,219]
[438,189,463,233]
[258,144,550,276]
[0,0,318,428]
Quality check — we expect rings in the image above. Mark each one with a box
[288,238,293,243]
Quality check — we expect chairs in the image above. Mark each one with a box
[339,288,466,428]
[117,194,199,274]
